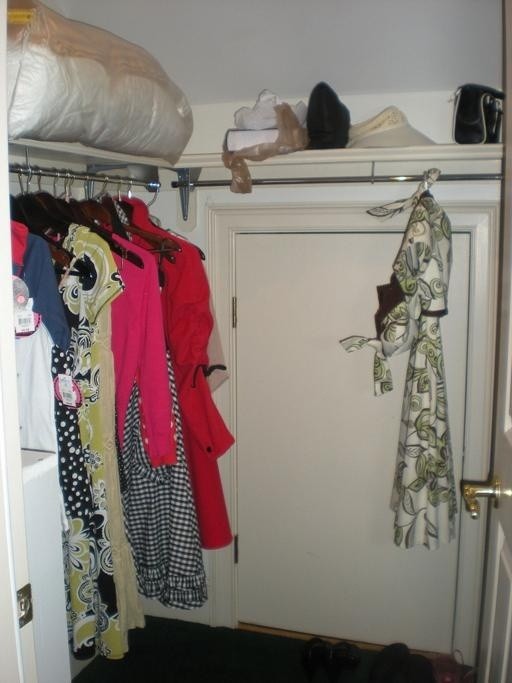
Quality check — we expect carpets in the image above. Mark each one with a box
[71,614,433,682]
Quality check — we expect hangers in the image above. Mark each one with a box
[411,172,447,224]
[14,163,205,277]
